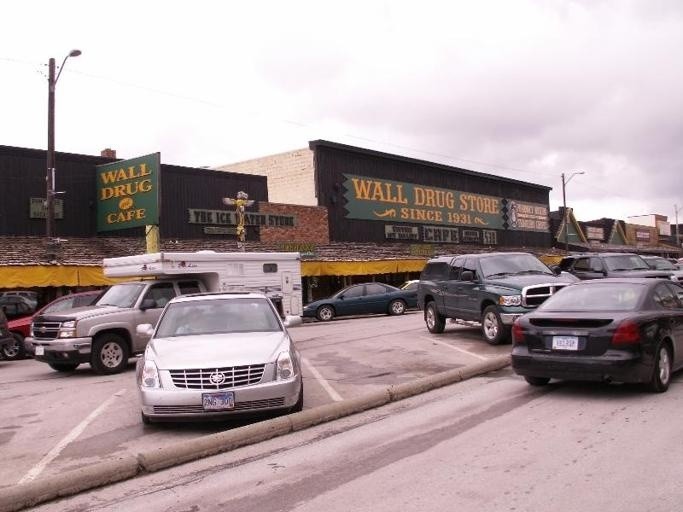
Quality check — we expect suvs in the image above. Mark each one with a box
[417,254,682,345]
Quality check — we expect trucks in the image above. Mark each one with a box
[23,250,303,375]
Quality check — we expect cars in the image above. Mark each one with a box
[304,280,418,321]
[510,276,682,392]
[135,291,303,423]
[0,290,102,361]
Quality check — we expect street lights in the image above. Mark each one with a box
[560,172,584,254]
[44,49,82,237]
